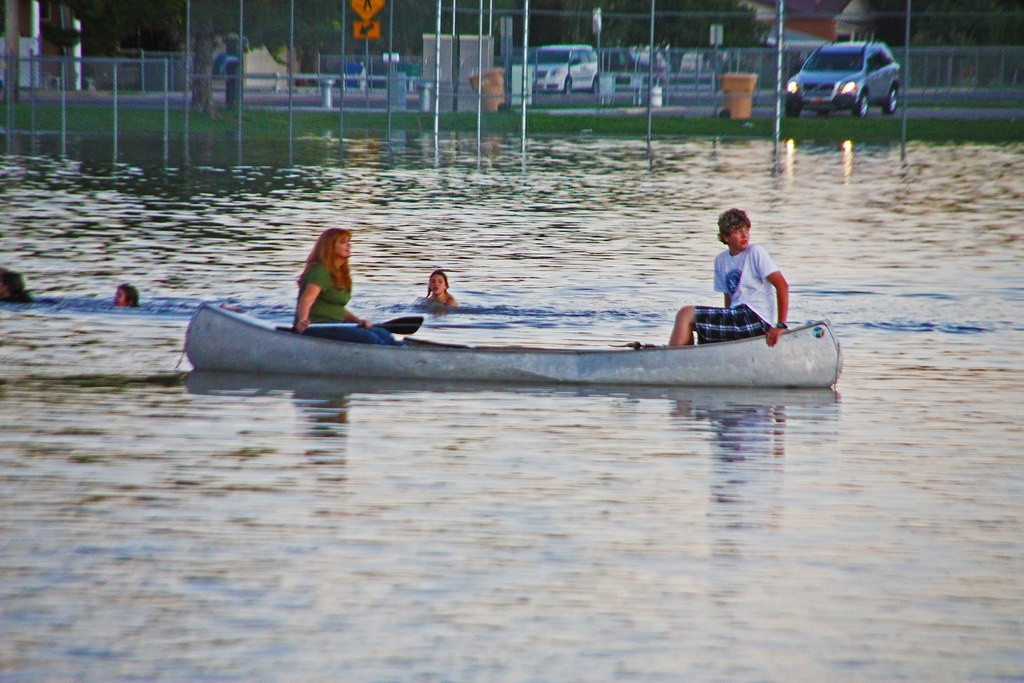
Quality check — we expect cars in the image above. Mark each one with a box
[601,44,729,92]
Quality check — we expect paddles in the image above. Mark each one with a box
[277,316,424,335]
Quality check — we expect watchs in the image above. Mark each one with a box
[776,321,789,330]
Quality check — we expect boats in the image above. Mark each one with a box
[183,298,844,391]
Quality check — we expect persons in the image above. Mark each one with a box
[292,228,402,347]
[425,270,459,307]
[665,207,789,348]
[0,270,33,304]
[114,283,139,308]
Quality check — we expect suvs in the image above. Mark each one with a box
[784,37,901,118]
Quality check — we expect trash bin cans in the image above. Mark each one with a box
[468,66,509,114]
[716,72,761,122]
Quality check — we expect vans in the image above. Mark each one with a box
[522,44,599,95]
